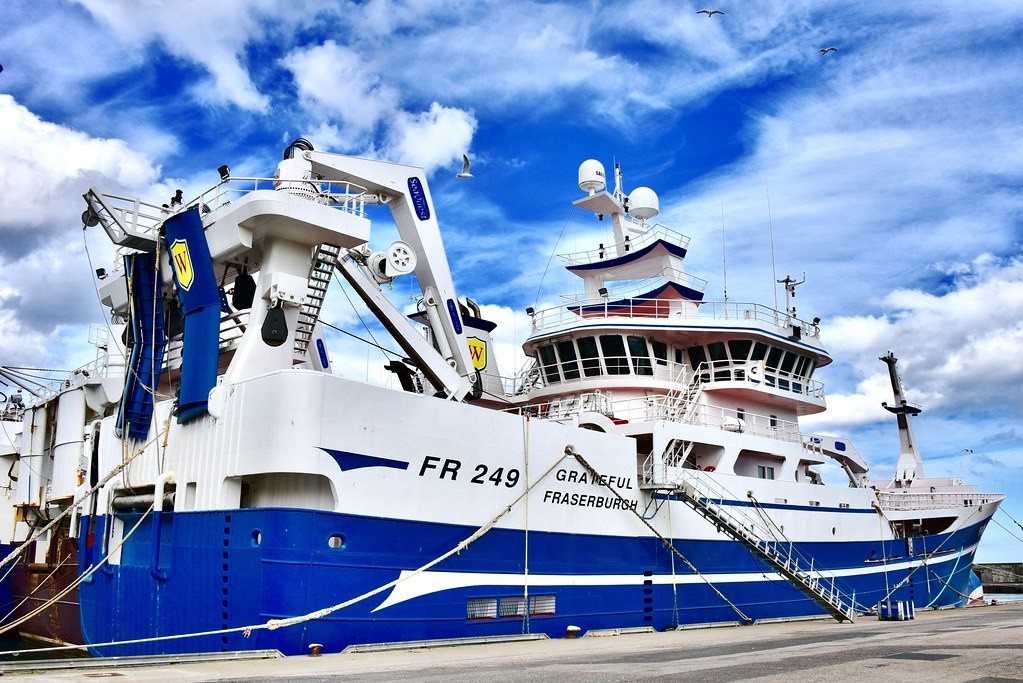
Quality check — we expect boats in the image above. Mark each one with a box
[1,139,1007,657]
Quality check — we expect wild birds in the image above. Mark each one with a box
[455,154,474,179]
[816,47,838,55]
[696,9,726,18]
